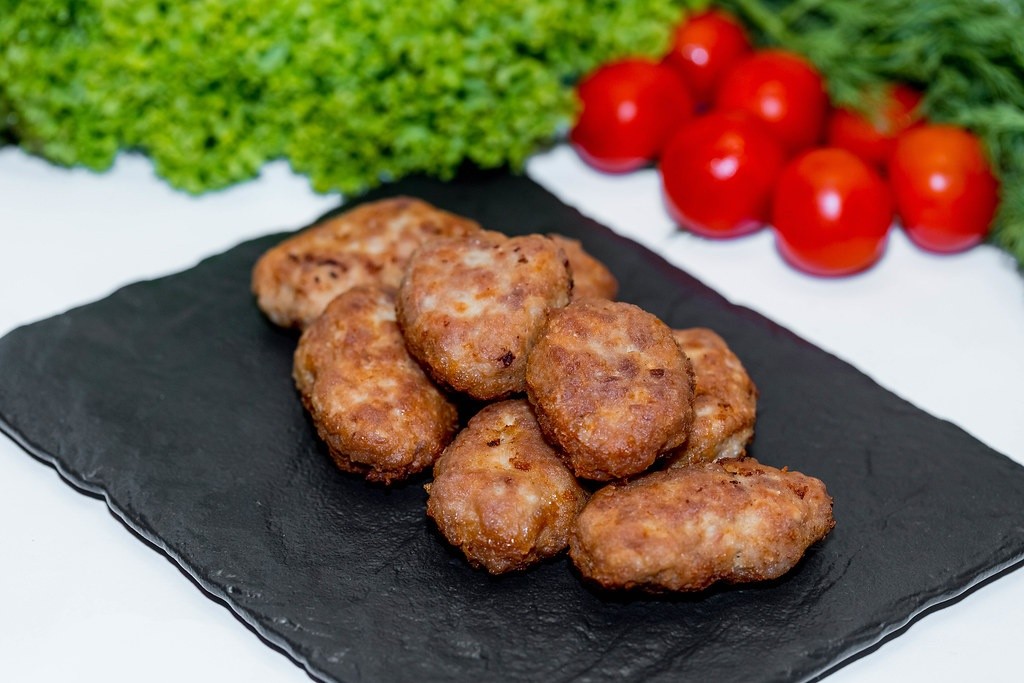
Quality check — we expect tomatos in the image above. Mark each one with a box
[569,21,995,276]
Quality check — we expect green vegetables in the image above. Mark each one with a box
[730,0,1024,273]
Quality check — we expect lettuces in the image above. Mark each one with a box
[0,0,712,194]
